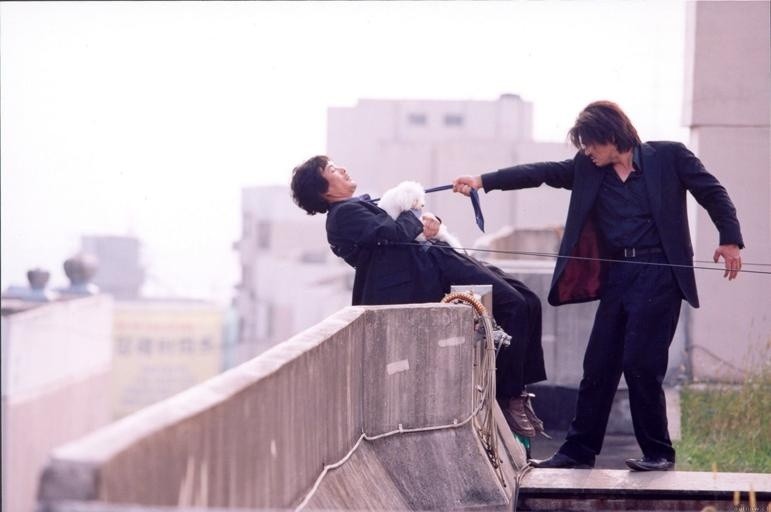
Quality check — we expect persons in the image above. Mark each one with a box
[452,98,746,472]
[292,156,546,438]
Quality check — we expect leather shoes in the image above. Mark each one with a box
[498,392,535,437]
[524,388,546,433]
[626,454,675,470]
[529,452,597,469]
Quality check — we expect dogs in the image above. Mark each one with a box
[377,181,468,257]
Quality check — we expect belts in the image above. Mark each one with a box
[606,246,662,259]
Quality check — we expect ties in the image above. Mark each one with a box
[366,184,484,232]
